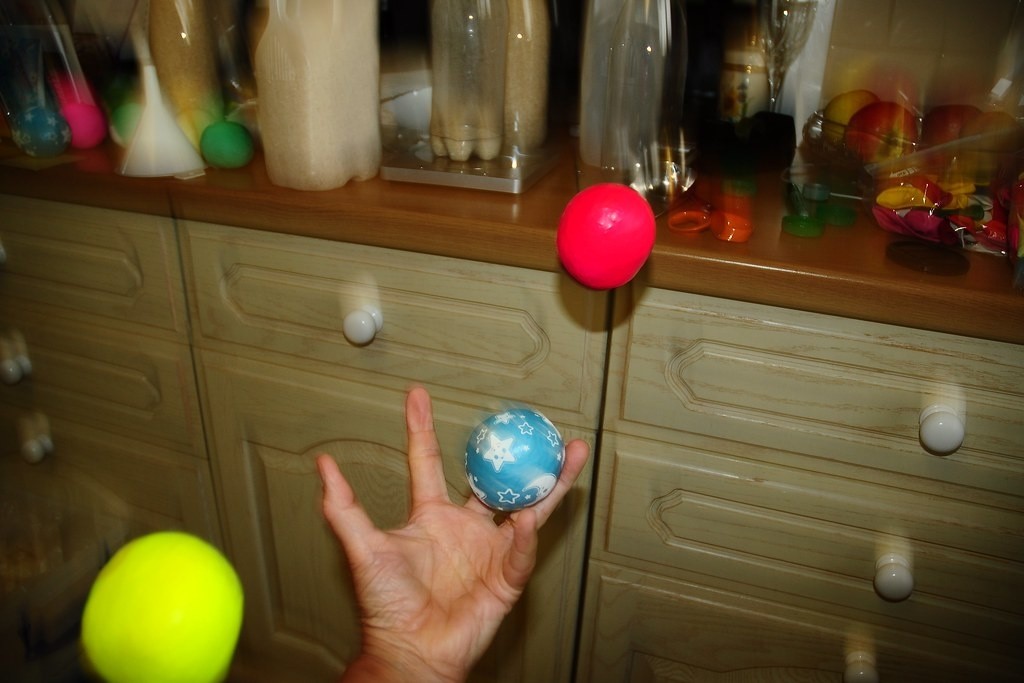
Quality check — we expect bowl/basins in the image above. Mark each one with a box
[802,108,1024,201]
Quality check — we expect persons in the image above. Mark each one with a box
[317,388,590,683]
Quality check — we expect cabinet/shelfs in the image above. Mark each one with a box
[0,192,225,682]
[173,215,609,682]
[573,278,1024,682]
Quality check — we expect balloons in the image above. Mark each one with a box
[874,169,1024,257]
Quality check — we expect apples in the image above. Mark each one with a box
[824,89,1021,183]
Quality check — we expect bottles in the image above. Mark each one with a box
[577,1,674,192]
[148,0,226,155]
[506,1,549,154]
[254,1,381,192]
[429,1,510,160]
